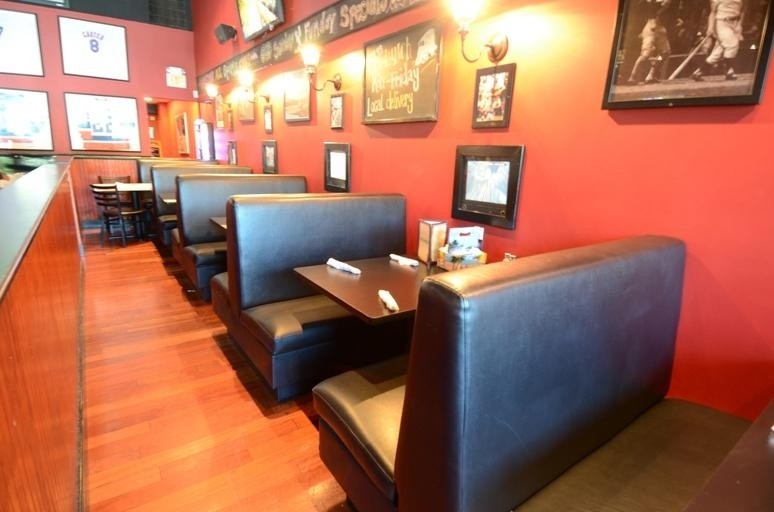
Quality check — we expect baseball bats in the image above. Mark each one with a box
[668,36,707,82]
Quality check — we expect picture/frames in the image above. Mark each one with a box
[264,105,274,134]
[0,88,54,151]
[174,112,189,153]
[58,15,129,82]
[330,95,343,129]
[65,92,143,152]
[283,69,311,122]
[227,141,236,165]
[0,8,44,77]
[361,18,442,125]
[323,142,350,192]
[261,140,278,174]
[238,86,255,122]
[471,63,516,129]
[452,145,524,228]
[601,0,774,110]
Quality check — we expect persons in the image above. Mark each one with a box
[476,73,510,122]
[615,0,767,86]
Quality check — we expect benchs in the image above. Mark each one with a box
[210,191,407,403]
[312,235,753,511]
[171,174,308,302]
[150,164,253,256]
[138,158,220,182]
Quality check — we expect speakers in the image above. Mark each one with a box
[214,23,236,44]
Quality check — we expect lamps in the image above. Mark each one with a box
[444,0,510,63]
[238,70,269,103]
[300,44,342,91]
[204,81,234,113]
[212,24,238,44]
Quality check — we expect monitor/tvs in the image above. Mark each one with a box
[461,155,510,217]
[264,146,275,171]
[326,148,346,188]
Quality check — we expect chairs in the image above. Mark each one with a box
[89,184,146,246]
[98,176,142,241]
